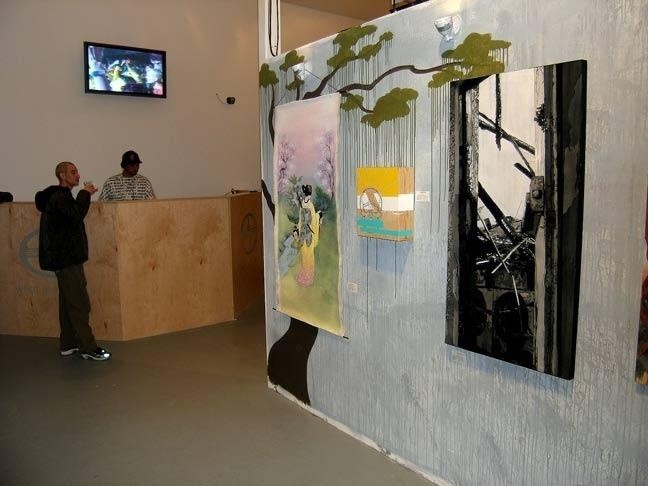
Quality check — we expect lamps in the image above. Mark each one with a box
[435,14,461,41]
[293,61,308,79]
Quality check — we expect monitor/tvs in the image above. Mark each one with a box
[84,41,166,98]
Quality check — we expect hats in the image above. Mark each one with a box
[122,150,142,165]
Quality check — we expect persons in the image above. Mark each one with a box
[99,151,156,201]
[279,226,300,279]
[35,162,112,362]
[297,185,320,287]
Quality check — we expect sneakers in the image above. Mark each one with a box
[81,346,110,361]
[60,345,80,357]
